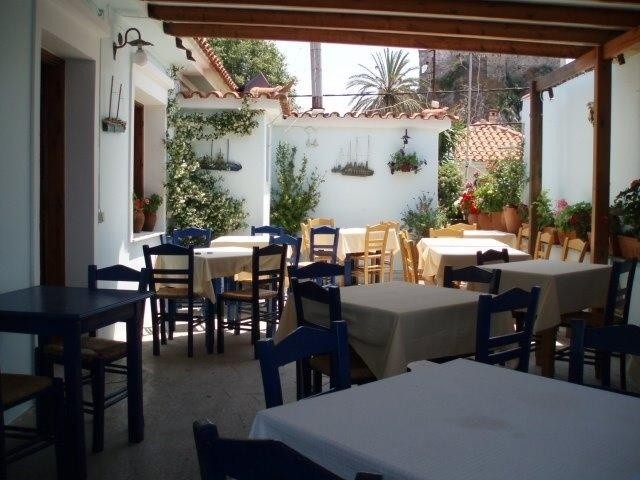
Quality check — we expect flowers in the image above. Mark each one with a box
[458,192,478,214]
[133,192,150,211]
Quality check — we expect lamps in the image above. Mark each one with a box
[549,87,554,98]
[113,28,153,66]
[401,129,410,148]
[618,53,626,65]
[585,102,594,126]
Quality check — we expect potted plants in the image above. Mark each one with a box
[388,150,427,174]
[532,179,640,262]
[473,154,529,233]
[142,193,163,231]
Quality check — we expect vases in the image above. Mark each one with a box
[467,214,476,225]
[134,208,145,233]
